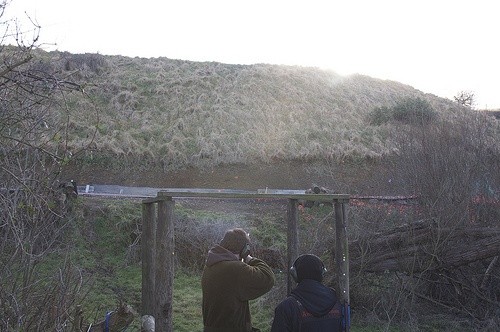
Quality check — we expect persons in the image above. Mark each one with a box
[270,254,350,332]
[201,228,276,332]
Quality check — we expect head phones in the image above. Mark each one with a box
[290,254,327,283]
[219,236,251,260]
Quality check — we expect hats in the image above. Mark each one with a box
[294,255,322,283]
